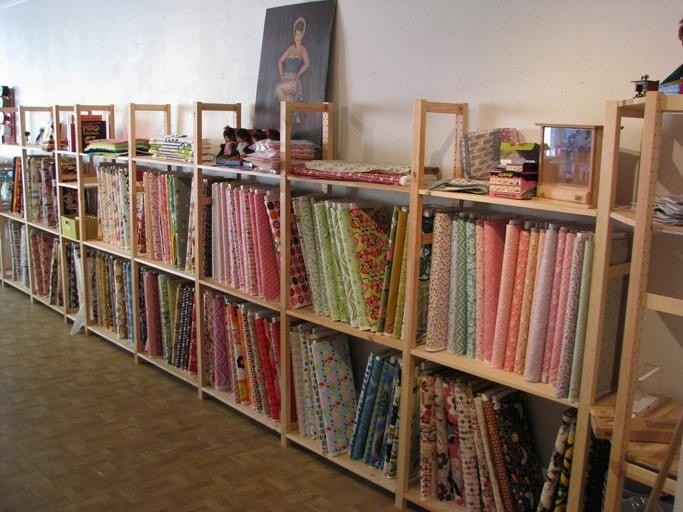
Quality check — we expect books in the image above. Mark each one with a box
[28,115,106,153]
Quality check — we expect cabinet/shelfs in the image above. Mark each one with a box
[400,96,608,512]
[53,104,88,329]
[128,100,200,388]
[192,99,287,434]
[573,95,683,512]
[1,108,31,297]
[278,99,419,494]
[19,105,66,316]
[74,103,137,357]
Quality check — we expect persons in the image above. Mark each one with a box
[275,17,310,103]
